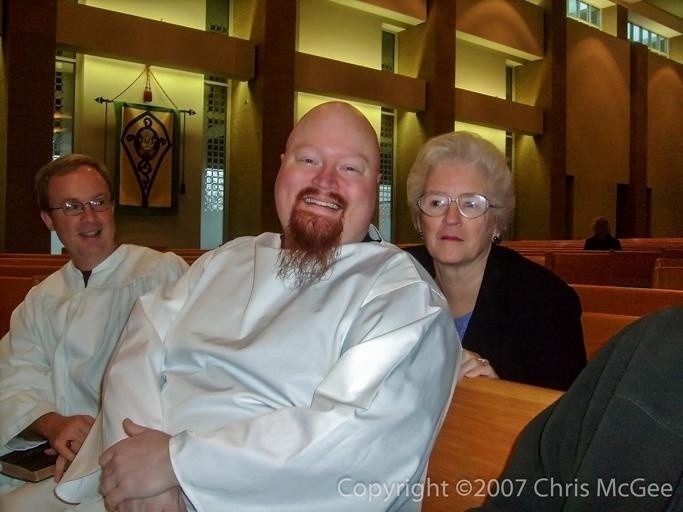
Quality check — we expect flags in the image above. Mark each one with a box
[94,60,197,216]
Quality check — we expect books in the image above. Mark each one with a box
[0,434,72,484]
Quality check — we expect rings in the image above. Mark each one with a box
[474,355,489,367]
[63,439,74,448]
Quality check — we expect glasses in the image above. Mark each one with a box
[417,191,500,219]
[47,196,114,217]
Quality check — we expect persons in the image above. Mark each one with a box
[55,101,463,512]
[582,218,623,252]
[395,132,589,396]
[0,155,189,510]
[463,297,683,512]
